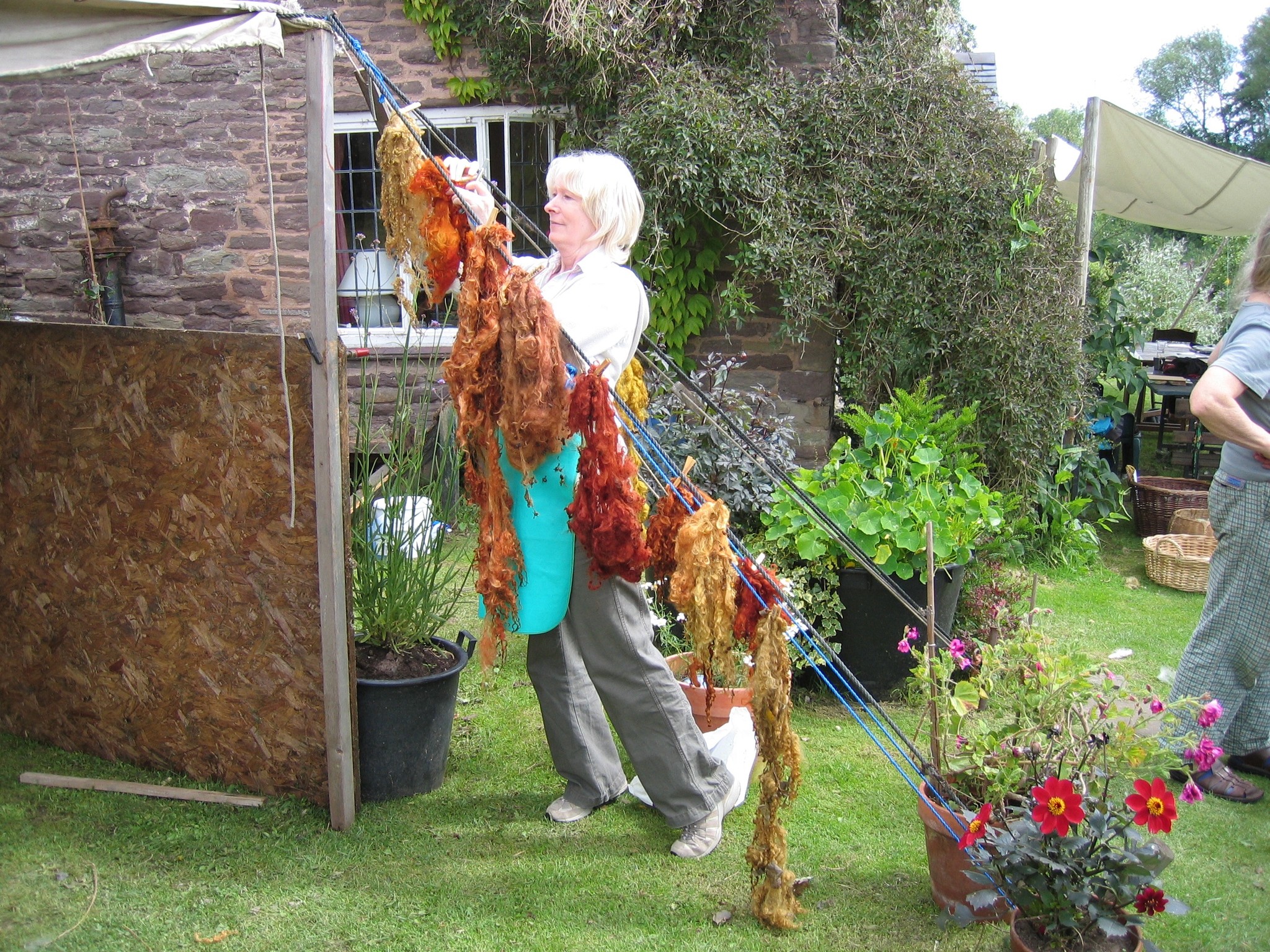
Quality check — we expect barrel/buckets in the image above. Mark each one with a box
[371,495,434,558]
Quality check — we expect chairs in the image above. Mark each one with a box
[1149,327,1198,409]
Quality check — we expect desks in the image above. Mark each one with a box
[1123,340,1216,411]
[1135,366,1201,456]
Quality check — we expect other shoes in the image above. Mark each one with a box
[1228,746,1270,778]
[1170,757,1265,803]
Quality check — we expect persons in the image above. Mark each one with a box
[428,149,739,861]
[1156,208,1270,805]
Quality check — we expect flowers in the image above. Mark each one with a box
[640,574,808,689]
[956,757,1191,952]
[339,232,478,677]
[896,603,1225,819]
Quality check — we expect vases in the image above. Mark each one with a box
[1009,893,1144,952]
[664,649,755,733]
[917,770,1014,926]
[354,628,478,802]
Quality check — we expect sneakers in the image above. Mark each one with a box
[670,779,741,859]
[545,777,628,822]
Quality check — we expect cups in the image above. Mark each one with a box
[1157,340,1166,353]
[1153,357,1166,371]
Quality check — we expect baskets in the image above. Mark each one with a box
[1142,534,1219,593]
[1126,464,1212,536]
[1167,508,1214,536]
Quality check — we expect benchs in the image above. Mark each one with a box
[1168,398,1226,481]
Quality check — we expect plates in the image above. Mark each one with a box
[1192,345,1214,353]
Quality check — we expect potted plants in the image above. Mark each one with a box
[756,408,1006,704]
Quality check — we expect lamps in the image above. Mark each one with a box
[337,248,400,327]
